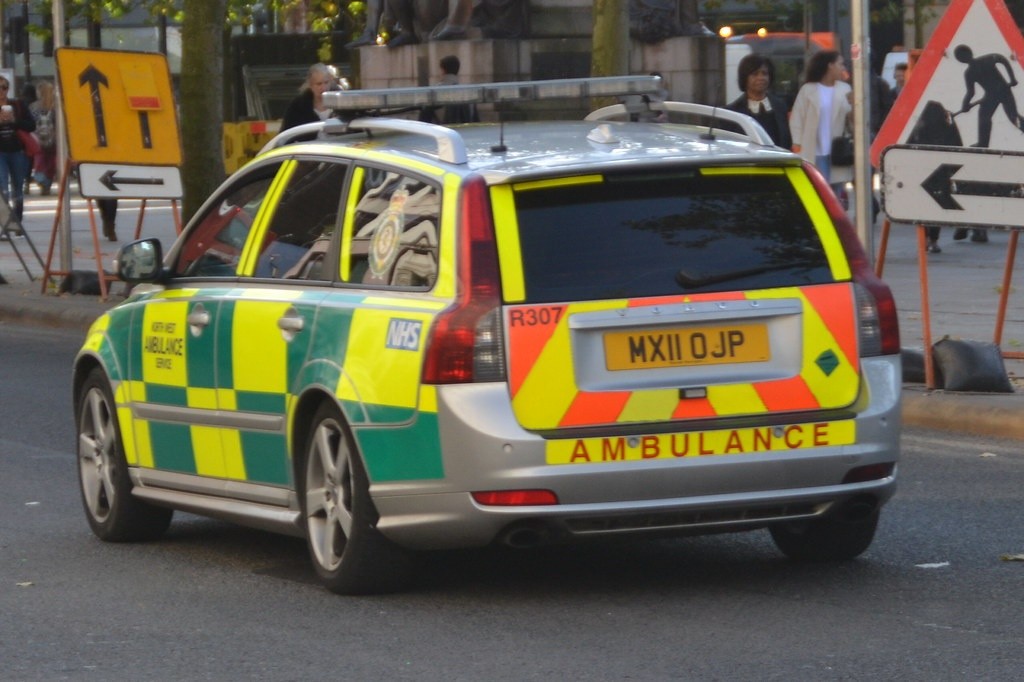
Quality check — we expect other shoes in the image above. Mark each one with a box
[953,230,968,241]
[972,234,988,242]
[924,243,941,254]
[103,223,117,242]
[1,234,10,243]
[16,226,25,238]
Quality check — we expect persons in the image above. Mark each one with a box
[417,55,482,125]
[28,80,62,194]
[788,48,856,214]
[280,61,355,146]
[952,226,991,244]
[889,62,915,107]
[924,224,943,254]
[98,200,119,241]
[0,76,36,241]
[718,50,794,154]
[14,82,39,193]
[866,49,894,226]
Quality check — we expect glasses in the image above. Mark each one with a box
[0,85,8,90]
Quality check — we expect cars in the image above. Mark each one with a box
[70,76,903,596]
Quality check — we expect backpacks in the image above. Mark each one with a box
[31,101,56,147]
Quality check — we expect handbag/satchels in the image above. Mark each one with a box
[831,115,854,166]
[15,99,40,156]
